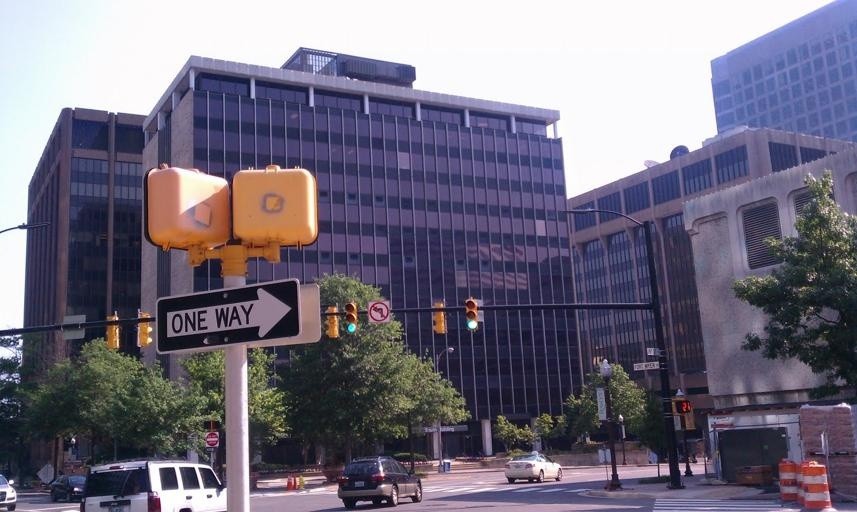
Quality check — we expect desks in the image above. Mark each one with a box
[0,220,51,235]
[562,208,686,489]
[70,438,75,461]
[435,346,455,473]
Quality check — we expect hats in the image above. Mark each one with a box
[504,454,564,483]
[0,474,17,511]
[337,457,422,508]
[50,475,86,503]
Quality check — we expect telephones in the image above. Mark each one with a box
[154,276,301,355]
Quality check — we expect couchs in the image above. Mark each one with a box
[675,398,692,414]
[105,312,153,349]
[432,297,479,334]
[324,302,357,338]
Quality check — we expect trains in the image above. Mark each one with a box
[204,431,219,448]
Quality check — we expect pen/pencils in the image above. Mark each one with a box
[79,459,227,512]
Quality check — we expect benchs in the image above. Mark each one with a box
[595,387,608,423]
